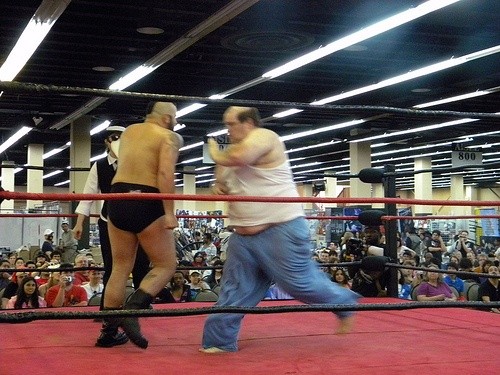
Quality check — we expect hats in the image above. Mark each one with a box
[193,252,204,258]
[213,259,224,265]
[44,229,54,236]
[364,224,381,232]
[60,220,69,225]
[105,119,128,132]
[188,269,201,276]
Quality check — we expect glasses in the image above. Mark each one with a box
[107,135,120,141]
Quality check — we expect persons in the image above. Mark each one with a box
[73,126,154,322]
[0,221,500,313]
[197,105,364,353]
[96,101,185,348]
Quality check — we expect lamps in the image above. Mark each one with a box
[0,0,500,191]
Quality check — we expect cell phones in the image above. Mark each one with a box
[198,277,200,283]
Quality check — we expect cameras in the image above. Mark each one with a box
[66,276,72,282]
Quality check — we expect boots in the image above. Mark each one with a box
[112,288,155,349]
[94,306,130,348]
